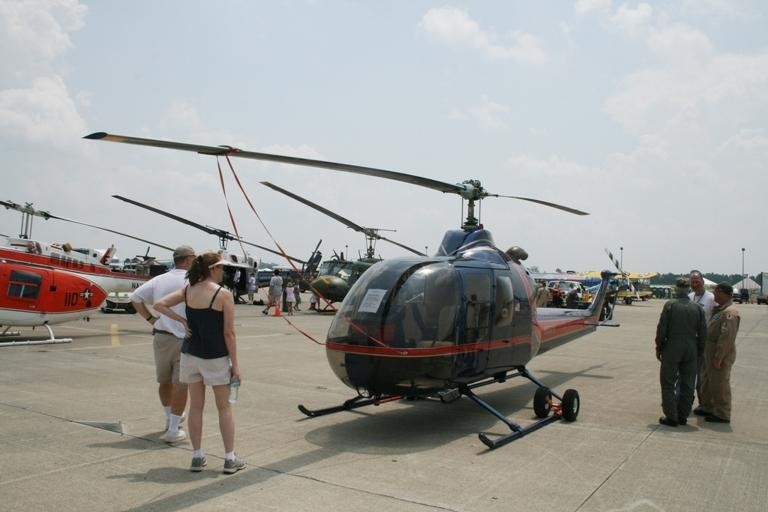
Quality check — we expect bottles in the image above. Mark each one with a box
[228,374,241,404]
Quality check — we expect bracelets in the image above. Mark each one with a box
[146,315,152,321]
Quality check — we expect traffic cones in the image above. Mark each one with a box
[272,301,282,317]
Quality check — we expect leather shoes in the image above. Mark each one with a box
[659,408,731,426]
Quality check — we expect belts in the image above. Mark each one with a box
[153,328,174,335]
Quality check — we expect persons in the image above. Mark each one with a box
[234,267,304,317]
[129,245,197,443]
[654,276,707,427]
[687,270,718,414]
[549,283,562,308]
[705,281,740,422]
[535,283,550,308]
[566,288,581,308]
[153,253,247,474]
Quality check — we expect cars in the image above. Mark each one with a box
[544,280,585,308]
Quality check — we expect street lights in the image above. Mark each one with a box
[741,248,746,289]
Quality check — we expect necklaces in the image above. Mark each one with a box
[694,290,706,302]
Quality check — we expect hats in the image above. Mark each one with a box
[710,282,733,296]
[675,276,691,290]
[172,245,197,258]
[208,258,232,269]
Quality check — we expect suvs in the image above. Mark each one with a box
[732,288,749,304]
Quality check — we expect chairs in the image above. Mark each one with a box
[418,300,476,375]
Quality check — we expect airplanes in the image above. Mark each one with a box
[583,268,658,306]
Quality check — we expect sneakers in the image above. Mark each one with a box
[189,455,207,471]
[163,412,188,443]
[221,457,247,474]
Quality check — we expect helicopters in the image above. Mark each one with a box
[255,179,433,312]
[111,194,310,305]
[0,200,177,350]
[82,131,631,452]
